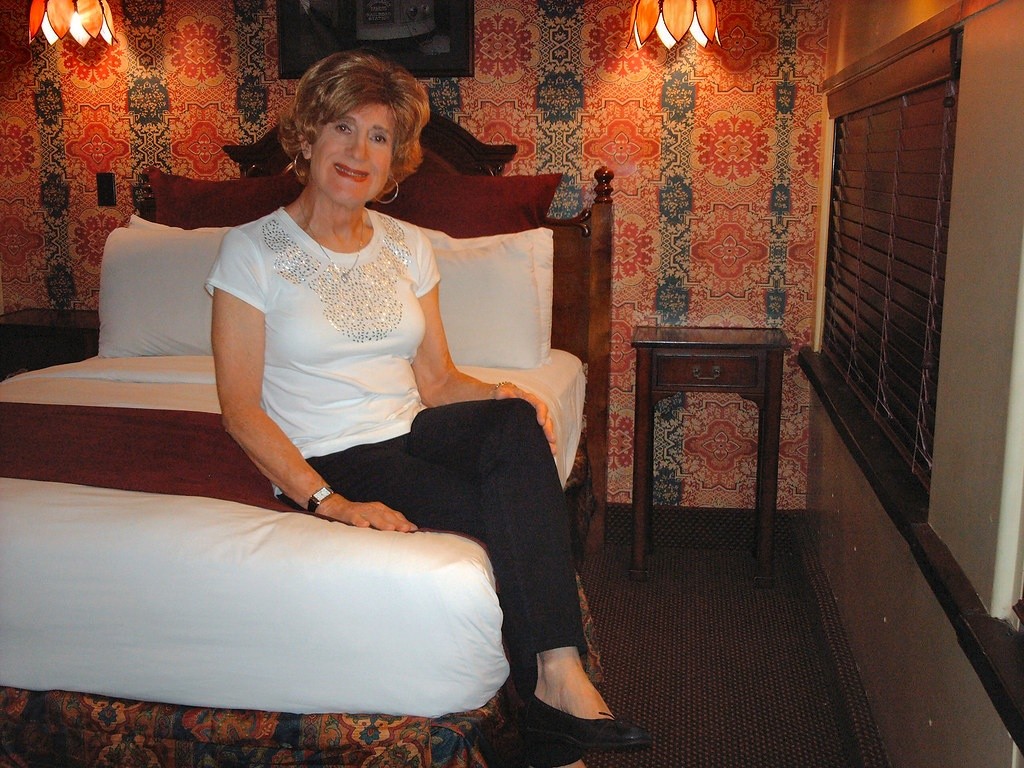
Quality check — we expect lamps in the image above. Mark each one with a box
[27,0,119,49]
[624,0,723,52]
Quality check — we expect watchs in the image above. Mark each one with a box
[306,485,334,516]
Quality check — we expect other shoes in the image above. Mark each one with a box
[515,701,585,768]
[525,693,652,752]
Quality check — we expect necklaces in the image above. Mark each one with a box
[297,196,363,282]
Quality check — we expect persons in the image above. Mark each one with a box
[202,54,653,768]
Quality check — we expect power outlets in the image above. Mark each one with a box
[97,173,116,206]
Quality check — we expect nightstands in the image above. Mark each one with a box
[630,327,790,589]
[0,308,101,384]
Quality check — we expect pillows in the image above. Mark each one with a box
[127,215,183,230]
[98,226,234,358]
[418,227,553,365]
[433,234,544,371]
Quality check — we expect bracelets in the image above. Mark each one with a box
[494,380,516,400]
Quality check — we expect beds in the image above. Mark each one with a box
[0,107,614,768]
